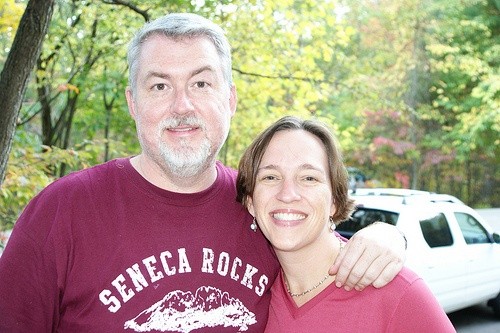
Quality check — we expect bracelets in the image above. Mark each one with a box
[374,221,408,251]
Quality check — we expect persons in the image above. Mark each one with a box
[235,114,457,333]
[0,12,406,333]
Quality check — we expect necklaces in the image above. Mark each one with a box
[283,274,330,297]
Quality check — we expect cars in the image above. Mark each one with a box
[330,196,500,317]
[344,169,467,205]
